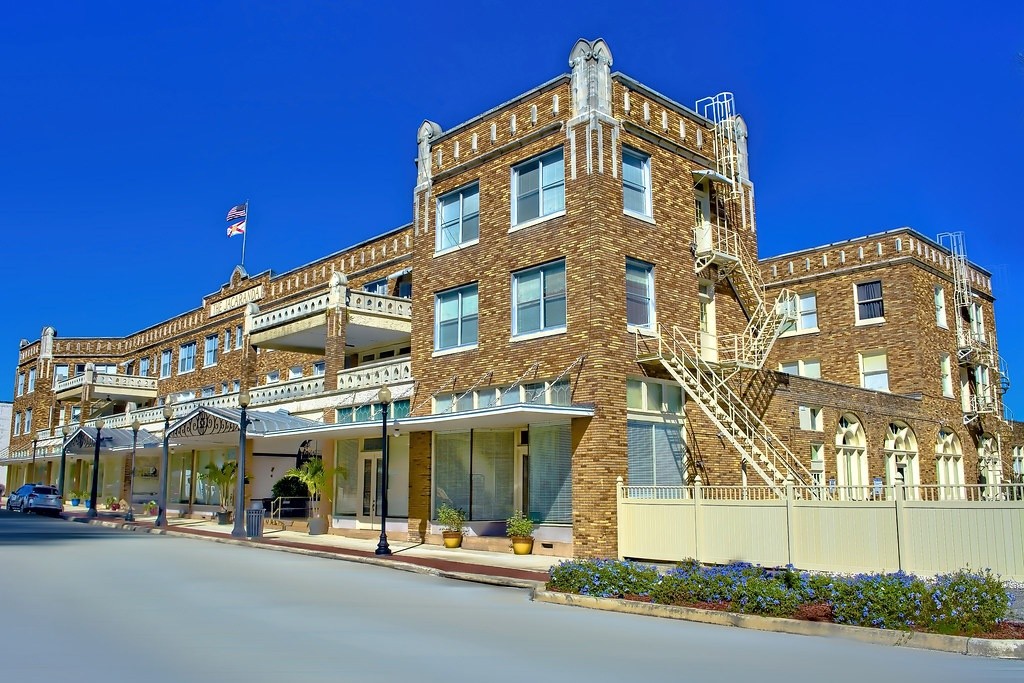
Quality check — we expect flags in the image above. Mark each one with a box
[226,203,246,222]
[226,220,245,238]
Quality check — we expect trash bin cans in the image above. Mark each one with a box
[245,508,266,538]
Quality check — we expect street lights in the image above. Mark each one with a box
[32,435,39,483]
[230,392,250,540]
[154,406,173,525]
[123,419,140,522]
[58,425,70,496]
[86,417,103,515]
[375,383,391,554]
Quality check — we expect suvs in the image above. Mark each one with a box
[6,483,63,517]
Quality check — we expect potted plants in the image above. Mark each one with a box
[68,490,80,506]
[81,491,91,508]
[197,462,254,524]
[506,509,534,555]
[146,501,158,515]
[286,454,347,536]
[435,503,467,547]
